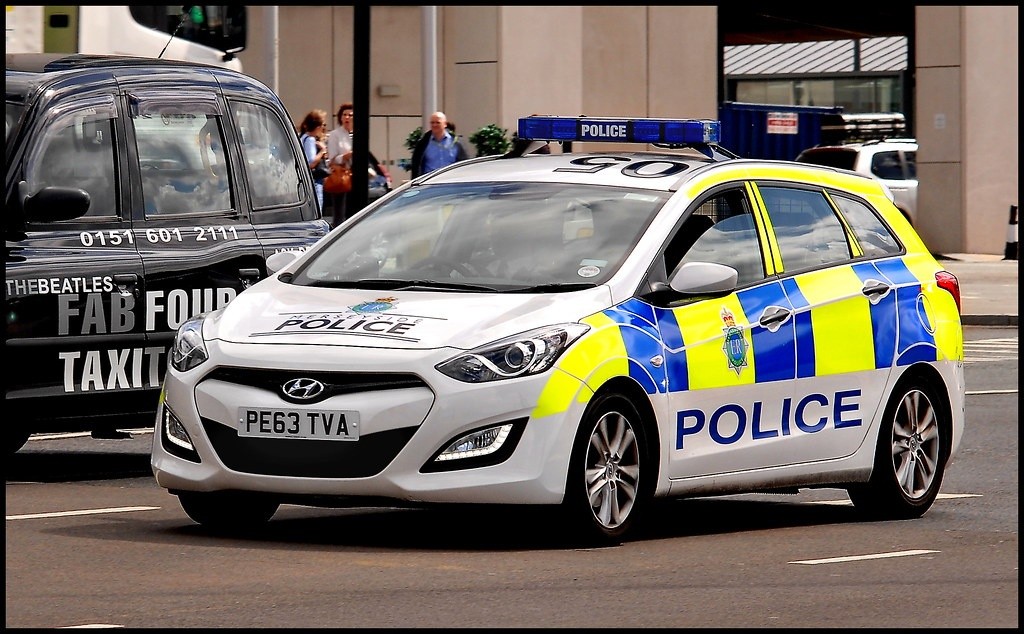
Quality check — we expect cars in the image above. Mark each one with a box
[149,112,963,542]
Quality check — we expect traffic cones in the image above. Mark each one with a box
[1002,208,1019,259]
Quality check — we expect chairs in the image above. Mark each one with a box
[481,214,824,283]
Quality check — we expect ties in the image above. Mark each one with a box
[496,260,507,279]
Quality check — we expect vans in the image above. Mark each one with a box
[794,138,918,227]
[3,53,331,466]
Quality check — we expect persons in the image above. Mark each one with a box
[411,112,468,180]
[299,102,354,227]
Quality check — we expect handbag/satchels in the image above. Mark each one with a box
[303,136,332,180]
[324,154,352,191]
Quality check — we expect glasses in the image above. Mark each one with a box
[322,123,327,126]
